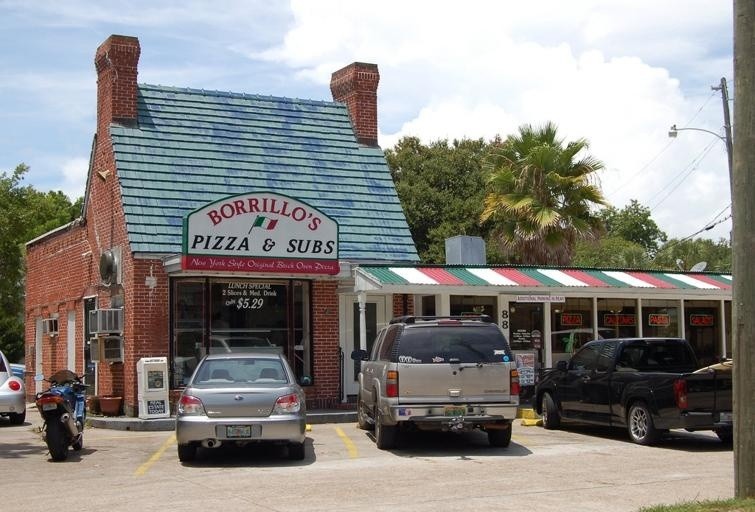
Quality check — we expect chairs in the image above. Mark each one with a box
[209,368,233,381]
[260,367,278,380]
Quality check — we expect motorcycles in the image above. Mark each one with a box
[31,369,90,460]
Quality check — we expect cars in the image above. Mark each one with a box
[1,348,30,428]
[173,350,311,459]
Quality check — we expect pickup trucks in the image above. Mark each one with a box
[531,334,732,449]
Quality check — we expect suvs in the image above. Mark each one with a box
[354,314,518,450]
